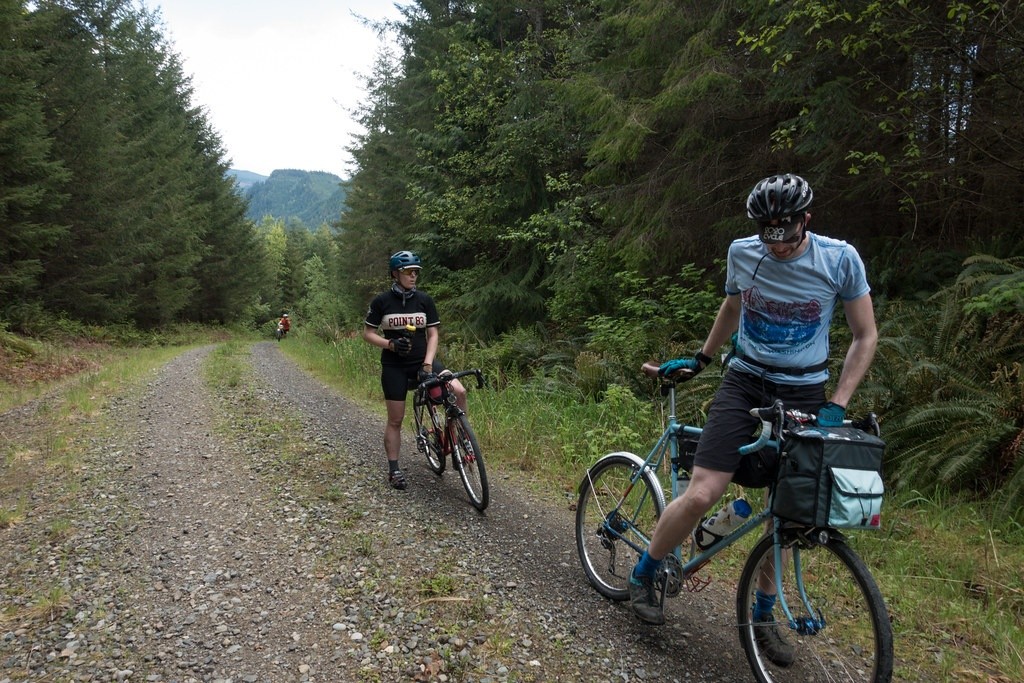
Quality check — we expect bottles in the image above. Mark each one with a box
[691,500,752,551]
[397,323,416,356]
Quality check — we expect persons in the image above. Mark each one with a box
[279,314,290,335]
[626,173,879,667]
[363,250,475,489]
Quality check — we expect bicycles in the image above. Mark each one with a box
[409,368,493,513]
[275,323,287,343]
[573,363,897,683]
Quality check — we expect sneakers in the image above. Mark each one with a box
[753,616,794,668]
[459,435,473,456]
[389,470,408,490]
[626,567,667,627]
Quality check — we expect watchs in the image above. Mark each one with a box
[695,349,712,366]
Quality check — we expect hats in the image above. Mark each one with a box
[399,262,423,272]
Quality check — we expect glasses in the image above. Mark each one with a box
[759,222,805,244]
[402,268,421,276]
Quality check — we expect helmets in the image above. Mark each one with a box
[389,251,421,272]
[283,314,288,317]
[747,173,815,233]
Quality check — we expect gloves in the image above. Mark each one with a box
[417,368,432,382]
[657,352,711,384]
[816,401,843,429]
[390,338,412,356]
